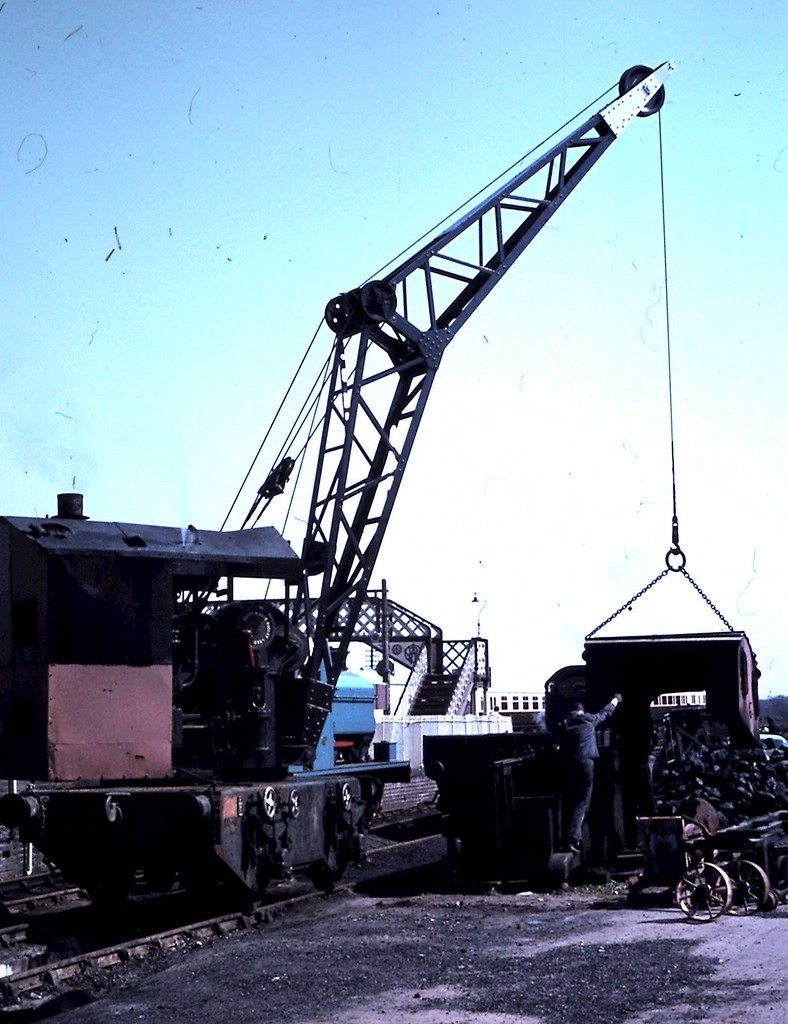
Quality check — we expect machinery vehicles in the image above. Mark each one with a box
[0,54,762,950]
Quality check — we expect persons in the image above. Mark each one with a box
[559,693,622,852]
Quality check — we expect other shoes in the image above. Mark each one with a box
[571,837,583,858]
